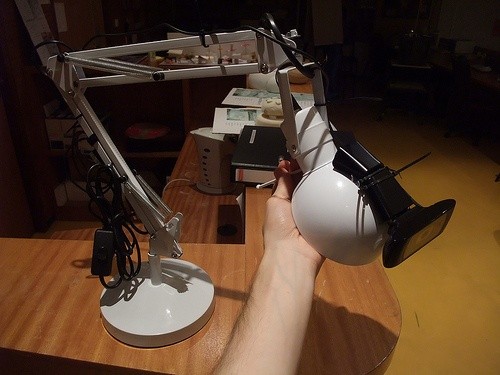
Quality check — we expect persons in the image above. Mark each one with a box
[211,158,327,375]
